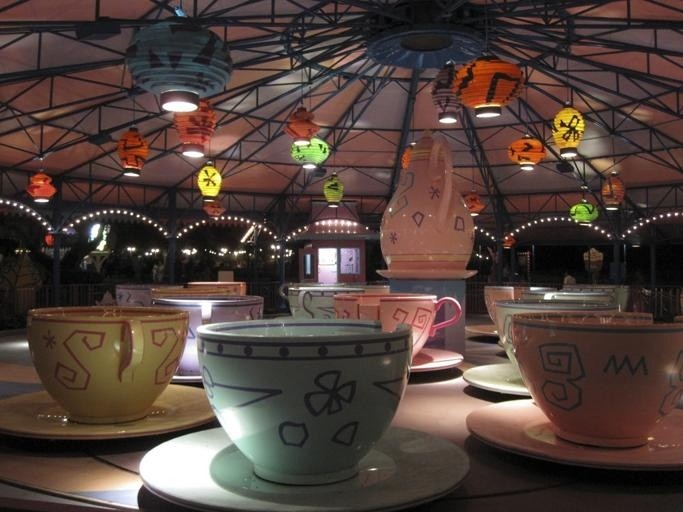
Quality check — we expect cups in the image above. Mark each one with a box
[279,281,463,359]
[23,304,191,425]
[193,315,415,489]
[114,280,265,377]
[482,283,683,452]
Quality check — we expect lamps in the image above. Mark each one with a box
[463,156,485,217]
[550,48,585,157]
[44,235,53,246]
[116,77,149,178]
[200,201,226,218]
[502,234,517,249]
[26,124,56,204]
[507,67,545,171]
[451,18,524,120]
[323,129,343,209]
[284,43,320,146]
[401,103,418,171]
[599,111,624,213]
[430,61,460,127]
[290,70,331,171]
[125,0,233,117]
[196,138,221,203]
[568,159,598,227]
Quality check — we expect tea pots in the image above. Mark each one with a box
[377,133,477,269]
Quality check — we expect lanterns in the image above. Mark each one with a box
[401,145,414,171]
[431,62,463,124]
[451,56,525,118]
[552,105,586,157]
[45,232,54,247]
[283,107,320,144]
[197,164,223,203]
[26,171,58,204]
[290,137,331,169]
[569,200,599,224]
[117,126,150,178]
[322,174,344,208]
[125,15,234,113]
[602,173,626,210]
[463,192,488,216]
[502,234,516,250]
[202,199,226,219]
[507,136,546,171]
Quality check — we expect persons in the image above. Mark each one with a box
[563,271,576,285]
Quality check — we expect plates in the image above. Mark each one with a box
[0,383,218,443]
[137,425,474,512]
[464,398,683,472]
[461,362,532,397]
[409,347,465,373]
[464,324,499,337]
[375,268,479,281]
